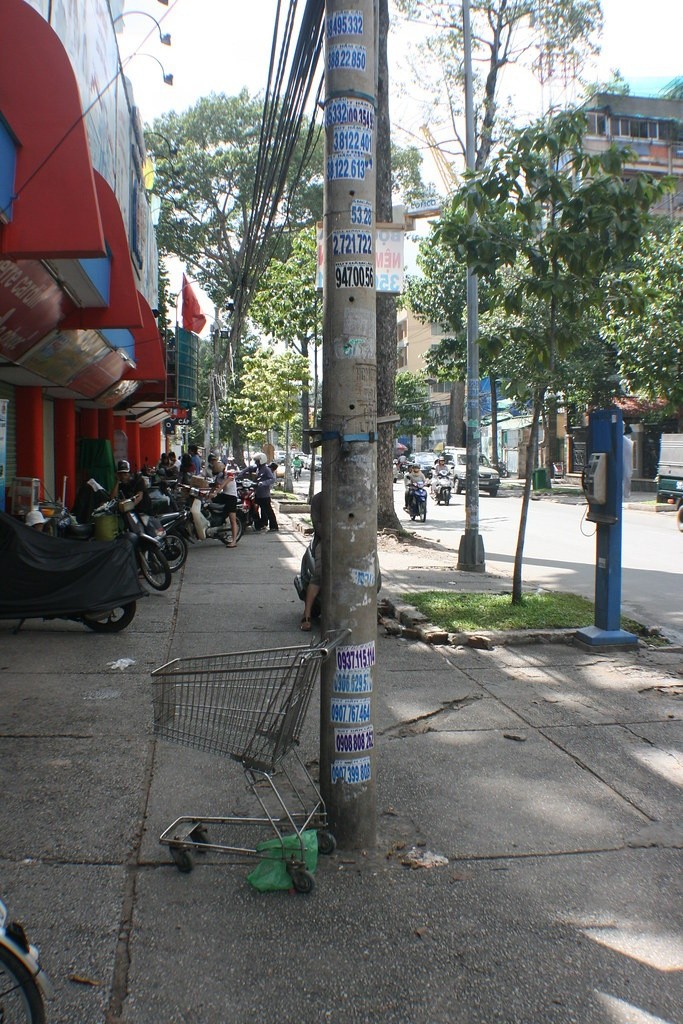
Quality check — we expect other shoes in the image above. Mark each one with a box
[226,543,237,547]
[156,528,166,537]
[268,528,278,532]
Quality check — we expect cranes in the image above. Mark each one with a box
[419,124,461,198]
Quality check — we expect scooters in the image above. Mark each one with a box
[430,466,454,506]
[294,540,381,618]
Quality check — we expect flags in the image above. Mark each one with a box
[181,274,206,333]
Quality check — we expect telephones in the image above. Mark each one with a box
[582,453,607,505]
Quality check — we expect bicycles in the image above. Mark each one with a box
[0,901,53,1024]
[291,465,304,481]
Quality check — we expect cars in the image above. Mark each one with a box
[276,451,286,463]
[276,466,285,478]
[291,452,322,472]
[407,452,439,479]
[440,446,501,497]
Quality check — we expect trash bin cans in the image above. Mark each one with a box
[534,468,547,489]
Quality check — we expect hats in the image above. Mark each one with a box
[24,510,51,527]
[117,460,130,473]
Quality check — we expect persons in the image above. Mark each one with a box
[300,491,323,631]
[109,442,302,548]
[392,455,454,521]
[25,510,50,533]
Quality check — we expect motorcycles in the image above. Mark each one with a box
[38,465,265,591]
[0,511,149,635]
[403,473,432,523]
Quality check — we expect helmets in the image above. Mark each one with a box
[412,463,421,469]
[268,462,278,470]
[207,454,218,463]
[435,458,440,464]
[408,464,414,470]
[215,462,226,473]
[253,453,267,464]
[439,457,445,461]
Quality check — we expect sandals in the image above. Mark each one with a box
[300,617,312,631]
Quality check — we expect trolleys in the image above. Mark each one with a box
[150,626,352,894]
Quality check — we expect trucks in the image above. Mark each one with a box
[655,434,683,533]
